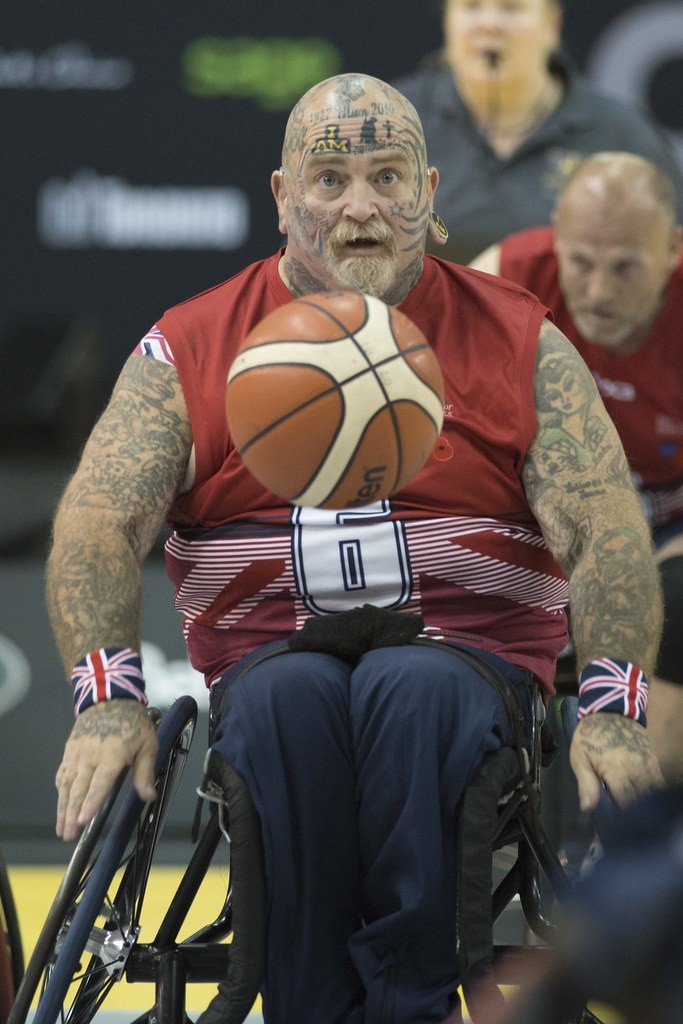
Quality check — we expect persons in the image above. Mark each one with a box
[394,0,683,264]
[468,150,683,846]
[45,73,660,1024]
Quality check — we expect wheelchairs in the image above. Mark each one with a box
[0,690,617,1023]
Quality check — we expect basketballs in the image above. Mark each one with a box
[221,291,449,509]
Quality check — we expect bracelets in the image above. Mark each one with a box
[576,657,648,728]
[69,645,150,719]
